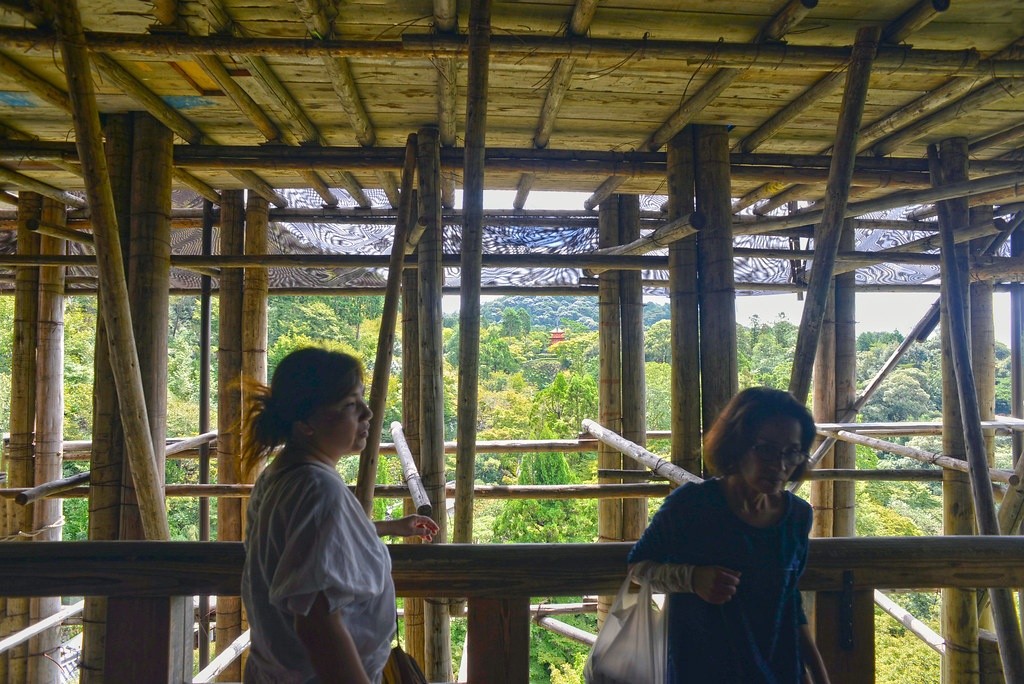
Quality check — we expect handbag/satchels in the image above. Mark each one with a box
[582,562,668,683]
[382,646,427,684]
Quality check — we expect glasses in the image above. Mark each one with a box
[751,438,810,467]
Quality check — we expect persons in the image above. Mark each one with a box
[625,387,832,684]
[240,346,440,684]
